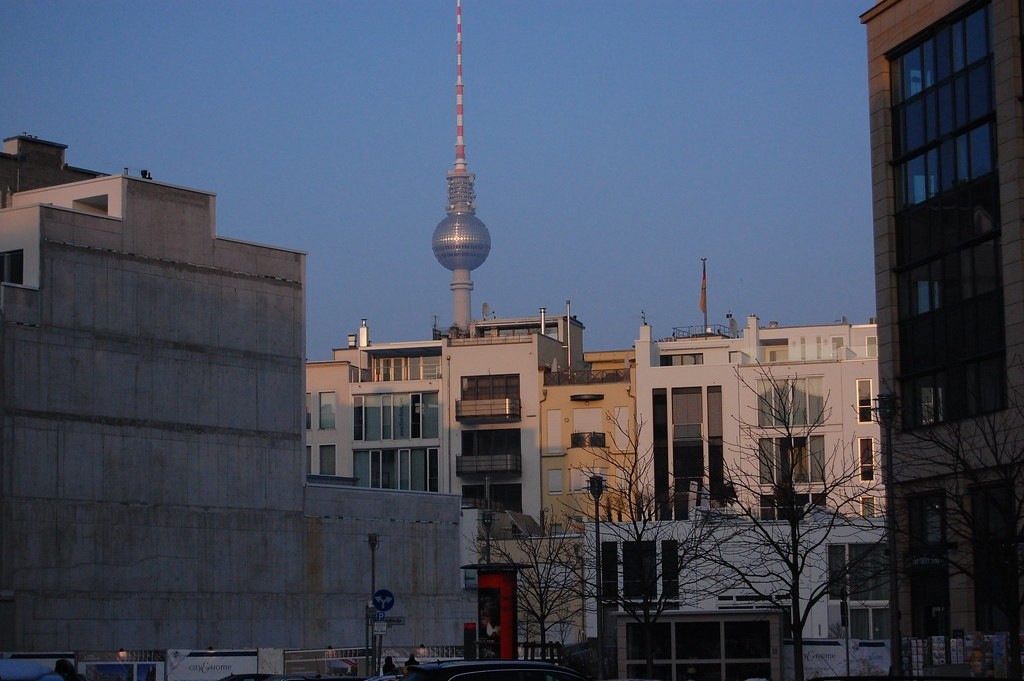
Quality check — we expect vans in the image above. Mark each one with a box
[402,657,598,681]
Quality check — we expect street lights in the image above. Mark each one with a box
[589,473,607,681]
[482,508,494,565]
[872,390,906,681]
[366,531,380,679]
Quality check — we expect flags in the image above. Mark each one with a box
[700,262,708,315]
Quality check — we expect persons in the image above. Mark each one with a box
[405,652,420,666]
[54,659,93,681]
[384,655,396,676]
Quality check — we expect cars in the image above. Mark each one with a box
[220,671,311,681]
